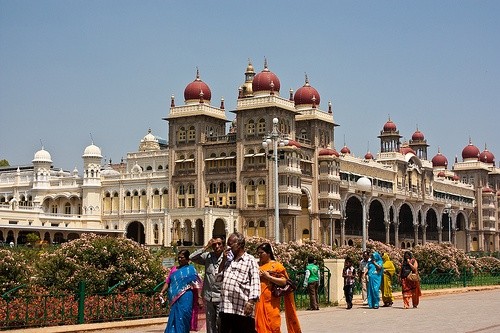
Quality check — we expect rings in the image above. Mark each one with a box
[261,273,262,275]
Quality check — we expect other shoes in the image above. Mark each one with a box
[404,305,409,309]
[374,306,378,309]
[307,308,319,311]
[413,306,417,308]
[383,303,392,307]
[347,303,353,309]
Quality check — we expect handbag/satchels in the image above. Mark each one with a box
[272,272,297,297]
[407,271,421,282]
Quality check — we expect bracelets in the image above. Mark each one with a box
[247,301,254,306]
[159,293,163,295]
[197,295,202,299]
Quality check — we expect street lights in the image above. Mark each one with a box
[262,117,290,242]
[356,177,372,251]
[443,204,455,242]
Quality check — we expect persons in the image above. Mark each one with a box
[359,246,396,309]
[342,257,356,310]
[189,235,230,333]
[159,250,204,333]
[217,232,261,333]
[10,238,71,249]
[303,256,321,311]
[400,251,422,309]
[255,243,303,333]
